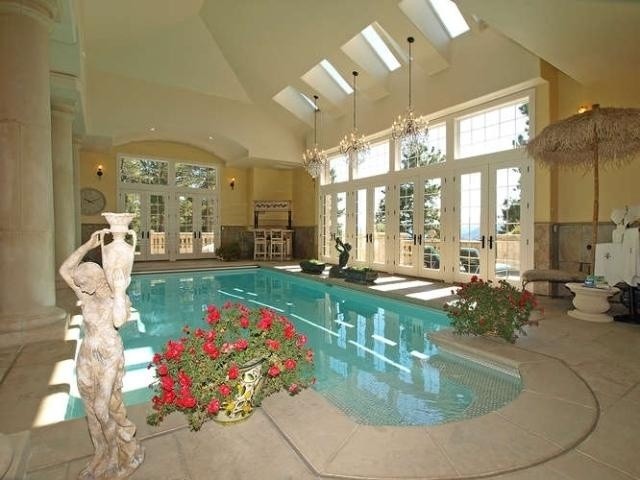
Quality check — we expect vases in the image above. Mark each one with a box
[202,354,273,425]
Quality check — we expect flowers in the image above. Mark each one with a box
[443,274,545,345]
[146,297,317,432]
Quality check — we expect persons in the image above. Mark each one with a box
[58,229,145,478]
[335,237,352,268]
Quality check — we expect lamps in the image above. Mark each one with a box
[303,94,330,179]
[336,71,370,171]
[389,37,430,155]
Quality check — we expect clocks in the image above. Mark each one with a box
[80,187,107,217]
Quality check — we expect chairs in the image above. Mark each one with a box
[252,228,289,263]
[424,245,511,276]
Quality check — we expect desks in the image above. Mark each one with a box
[249,229,294,259]
[520,268,590,298]
